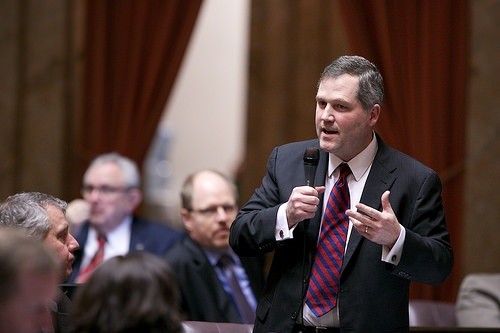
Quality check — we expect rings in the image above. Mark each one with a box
[364,226,368,233]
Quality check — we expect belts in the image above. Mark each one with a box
[303,325,340,333]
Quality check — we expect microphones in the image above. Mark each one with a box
[302,145,320,229]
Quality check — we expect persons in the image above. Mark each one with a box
[65,154,181,285]
[229,56,452,333]
[66,251,185,333]
[0,192,80,280]
[163,169,258,324]
[0,226,62,333]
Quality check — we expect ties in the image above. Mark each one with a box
[75,235,106,285]
[305,163,353,317]
[217,254,255,324]
